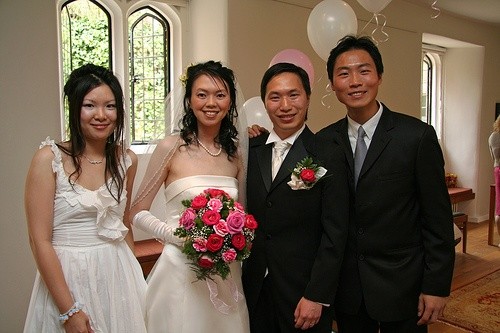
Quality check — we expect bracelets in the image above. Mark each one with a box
[58,301,81,324]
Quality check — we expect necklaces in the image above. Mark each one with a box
[192,130,223,156]
[83,152,105,163]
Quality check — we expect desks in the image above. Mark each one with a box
[487,183,496,246]
[448,186,476,202]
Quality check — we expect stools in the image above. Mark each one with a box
[452,211,468,254]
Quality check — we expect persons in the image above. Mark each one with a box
[245,35,456,333]
[239,61,347,333]
[488,114,500,247]
[129,61,251,333]
[23,62,148,333]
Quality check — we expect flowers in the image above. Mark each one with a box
[174,187,259,283]
[287,155,330,191]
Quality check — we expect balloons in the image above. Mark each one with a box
[307,0,358,62]
[357,0,392,14]
[269,49,314,91]
[237,96,274,147]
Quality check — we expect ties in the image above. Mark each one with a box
[265,140,291,277]
[354,125,368,190]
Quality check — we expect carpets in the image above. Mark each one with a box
[434,265,500,333]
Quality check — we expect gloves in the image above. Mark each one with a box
[132,210,189,247]
